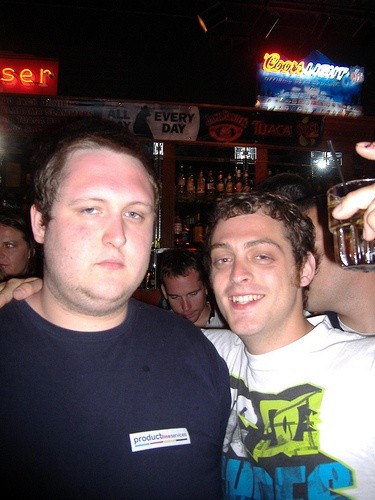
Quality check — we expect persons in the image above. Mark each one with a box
[157,248,229,328]
[256,172,375,338]
[0,133,375,500]
[0,208,36,282]
[0,133,232,500]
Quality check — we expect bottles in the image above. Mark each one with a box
[176,165,250,199]
[175,208,213,247]
[138,260,154,288]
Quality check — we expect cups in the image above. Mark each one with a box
[326,177,374,272]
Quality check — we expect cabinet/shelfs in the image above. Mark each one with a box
[159,141,269,297]
